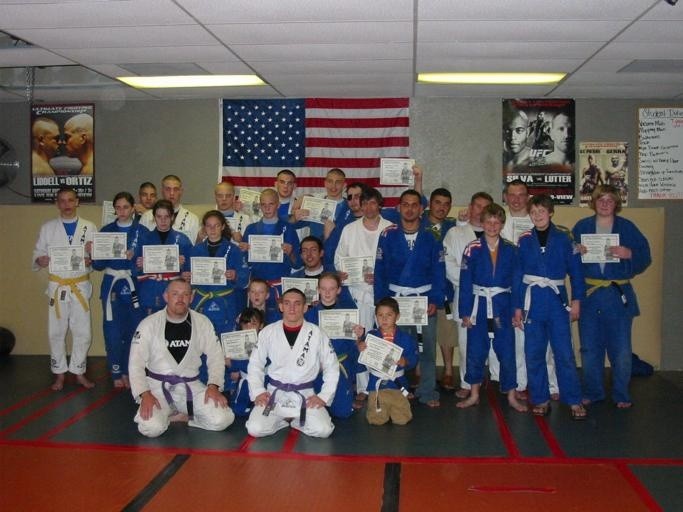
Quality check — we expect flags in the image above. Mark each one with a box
[217,97,414,210]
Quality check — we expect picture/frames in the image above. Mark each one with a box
[29,102,96,202]
[636,104,683,202]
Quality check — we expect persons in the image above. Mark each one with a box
[83,191,151,389]
[541,109,574,165]
[603,237,614,260]
[69,248,82,271]
[32,116,62,180]
[112,235,125,258]
[130,162,588,441]
[64,114,94,174]
[530,108,552,148]
[504,108,538,170]
[581,154,605,195]
[31,187,99,392]
[571,184,652,410]
[605,151,628,188]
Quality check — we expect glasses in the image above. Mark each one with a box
[301,249,318,253]
[402,203,419,208]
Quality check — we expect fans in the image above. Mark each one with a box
[0,138,19,189]
